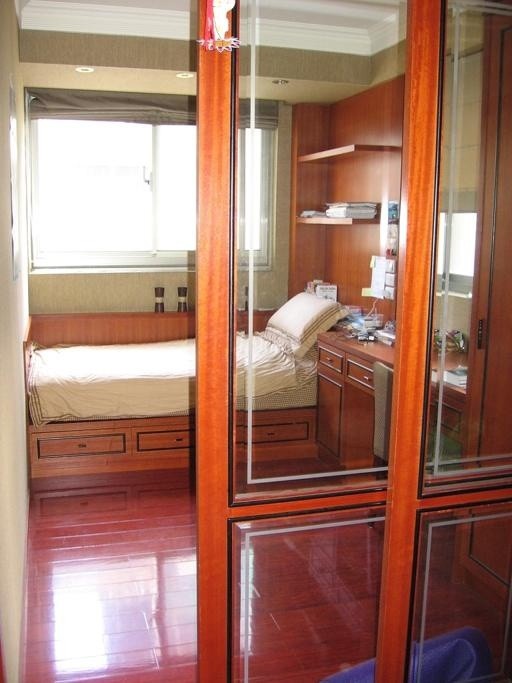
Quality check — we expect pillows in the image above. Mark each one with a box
[260,291,349,357]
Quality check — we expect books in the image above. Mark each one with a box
[432,364,467,389]
[306,279,337,301]
[300,200,378,219]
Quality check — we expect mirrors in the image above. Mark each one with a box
[433,190,479,352]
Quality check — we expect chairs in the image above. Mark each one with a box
[367,361,460,528]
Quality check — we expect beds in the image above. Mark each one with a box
[27,312,347,477]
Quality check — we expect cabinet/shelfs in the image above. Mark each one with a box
[288,74,405,333]
[453,12,512,614]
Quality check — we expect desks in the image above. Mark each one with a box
[313,325,470,483]
